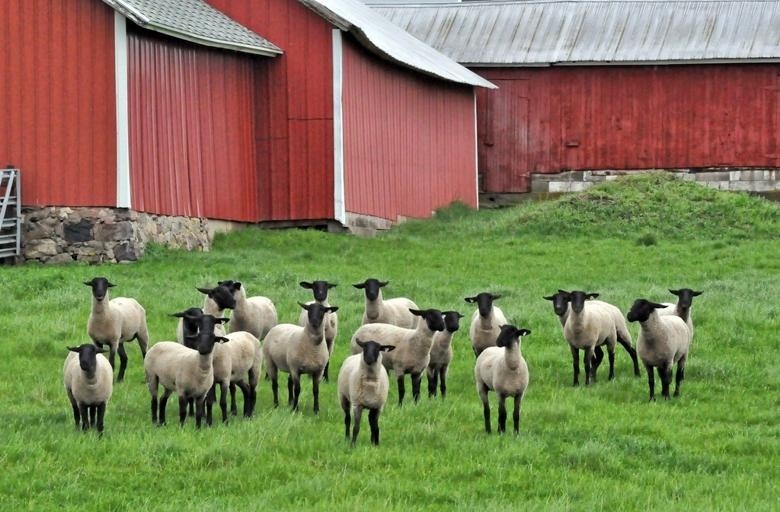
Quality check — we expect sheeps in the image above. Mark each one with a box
[351,278,421,330]
[82,277,149,383]
[350,308,446,407]
[474,324,532,437]
[542,289,704,403]
[338,338,395,451]
[143,279,340,431]
[427,311,465,400]
[63,343,114,440]
[464,292,508,358]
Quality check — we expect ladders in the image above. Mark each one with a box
[0,168,22,264]
